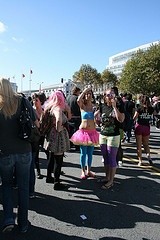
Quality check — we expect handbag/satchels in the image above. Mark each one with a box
[18,97,41,143]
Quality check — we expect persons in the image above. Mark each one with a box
[0,77,38,236]
[38,91,75,191]
[17,93,40,198]
[28,86,160,179]
[100,87,126,189]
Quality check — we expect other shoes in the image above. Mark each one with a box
[1,222,14,235]
[36,171,41,178]
[53,183,68,190]
[100,181,114,190]
[80,172,96,180]
[46,177,62,183]
[97,176,110,182]
